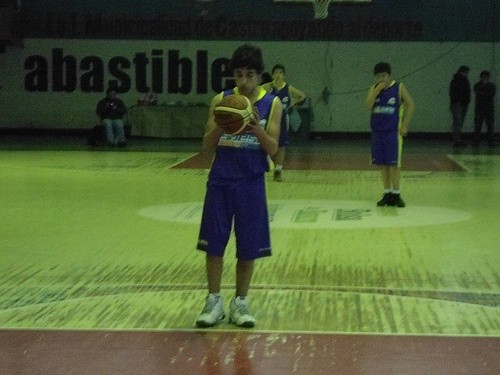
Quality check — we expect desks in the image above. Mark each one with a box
[130,104,208,138]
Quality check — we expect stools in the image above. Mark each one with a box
[94,123,132,144]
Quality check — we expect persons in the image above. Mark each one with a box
[449,64,472,147]
[195,44,284,329]
[365,62,415,206]
[259,63,305,181]
[96,86,128,147]
[471,69,496,147]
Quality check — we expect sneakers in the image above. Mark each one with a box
[197,293,227,328]
[229,296,256,327]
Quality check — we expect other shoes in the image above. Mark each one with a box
[377,192,405,207]
[274,168,282,181]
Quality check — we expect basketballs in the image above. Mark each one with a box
[212,94,257,136]
